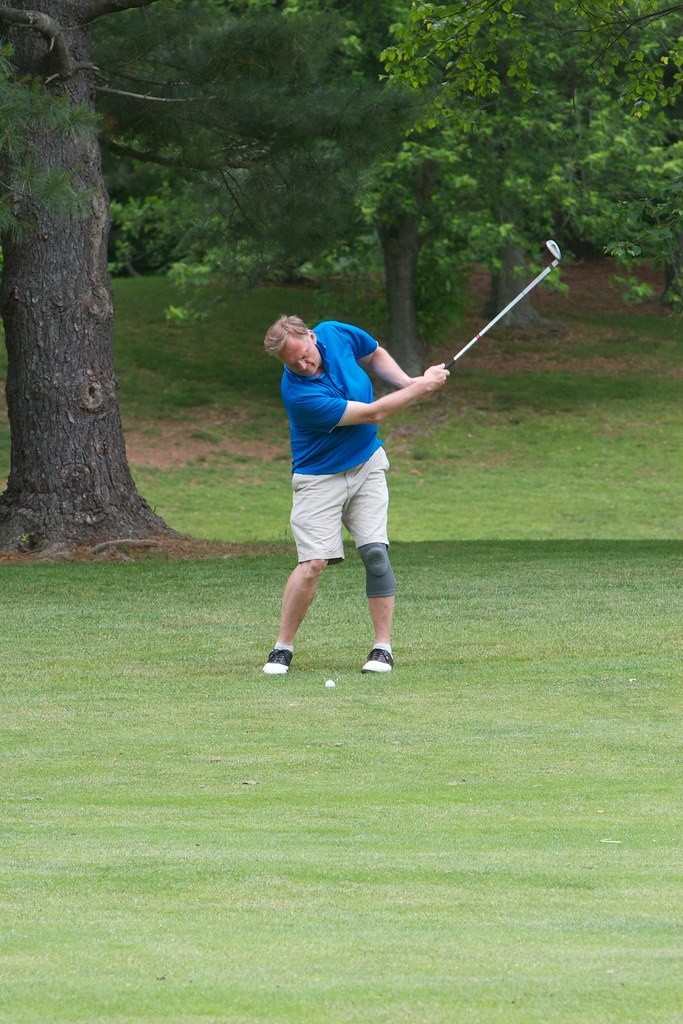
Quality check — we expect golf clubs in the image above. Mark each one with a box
[444,239,563,369]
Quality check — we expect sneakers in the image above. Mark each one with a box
[360,648,395,674]
[263,642,294,676]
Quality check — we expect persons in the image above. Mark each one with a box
[264,314,451,674]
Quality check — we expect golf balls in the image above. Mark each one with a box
[325,680,336,689]
